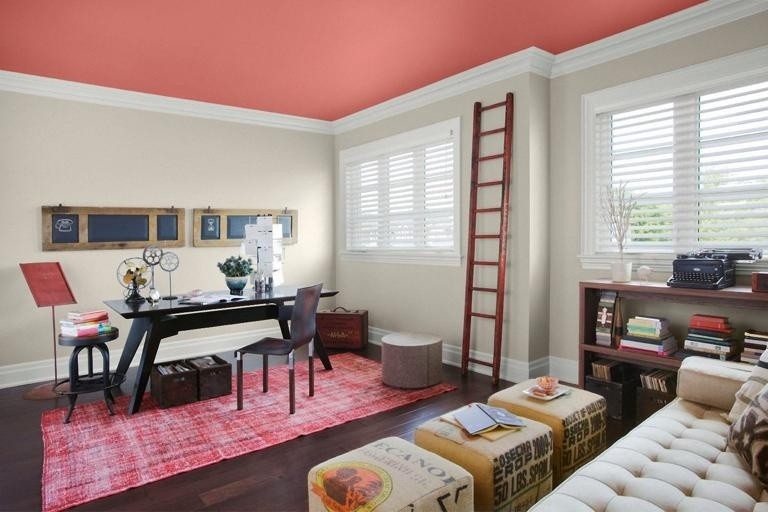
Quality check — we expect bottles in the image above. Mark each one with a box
[255,270,265,293]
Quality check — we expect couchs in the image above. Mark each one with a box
[526,354,768,512]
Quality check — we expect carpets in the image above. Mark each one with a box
[41,349,459,512]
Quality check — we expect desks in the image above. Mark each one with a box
[53,327,127,424]
[101,287,340,415]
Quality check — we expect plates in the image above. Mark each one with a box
[523,384,569,402]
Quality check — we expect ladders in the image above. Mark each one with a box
[461,92,514,385]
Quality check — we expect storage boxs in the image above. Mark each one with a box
[585,374,638,423]
[150,359,198,410]
[635,385,677,426]
[185,354,232,401]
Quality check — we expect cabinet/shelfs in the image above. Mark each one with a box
[578,280,768,388]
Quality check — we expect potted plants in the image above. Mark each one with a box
[586,181,639,282]
[216,255,254,296]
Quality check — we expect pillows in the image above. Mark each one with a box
[720,342,768,425]
[726,381,768,495]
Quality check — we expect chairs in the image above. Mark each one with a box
[235,283,324,414]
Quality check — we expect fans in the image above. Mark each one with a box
[118,257,153,304]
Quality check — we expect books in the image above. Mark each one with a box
[179,294,246,306]
[190,357,221,376]
[60,310,112,337]
[440,403,527,442]
[156,361,187,383]
[589,357,626,384]
[680,314,739,361]
[740,328,768,365]
[638,369,675,404]
[618,315,678,356]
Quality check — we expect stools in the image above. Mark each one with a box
[308,436,475,512]
[414,403,553,512]
[381,332,443,390]
[488,378,608,486]
